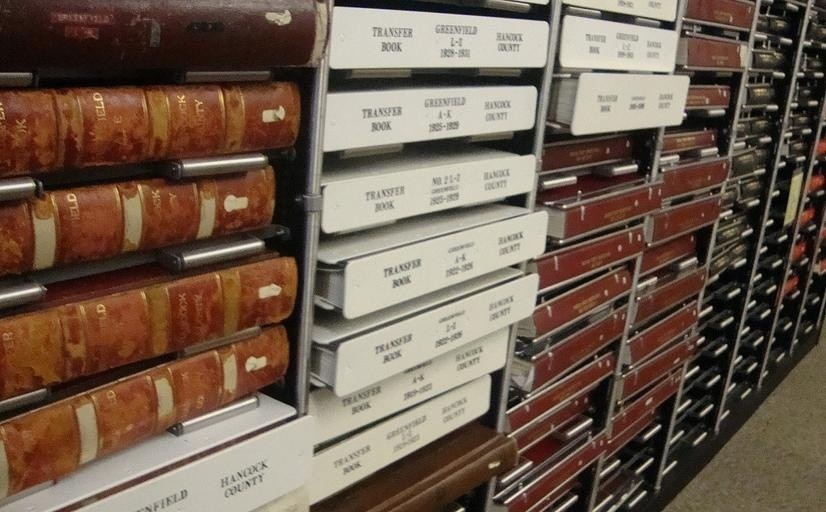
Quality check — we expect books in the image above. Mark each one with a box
[327,4,550,72]
[560,0,680,22]
[0,248,298,403]
[319,154,536,233]
[1,325,289,501]
[514,265,633,342]
[508,303,630,393]
[317,204,548,319]
[547,70,688,135]
[0,0,330,75]
[306,327,509,448]
[310,378,491,502]
[555,14,679,72]
[0,83,300,178]
[311,269,540,397]
[327,81,536,154]
[540,137,638,172]
[0,167,274,278]
[528,223,652,293]
[551,182,662,245]
[0,394,310,512]
